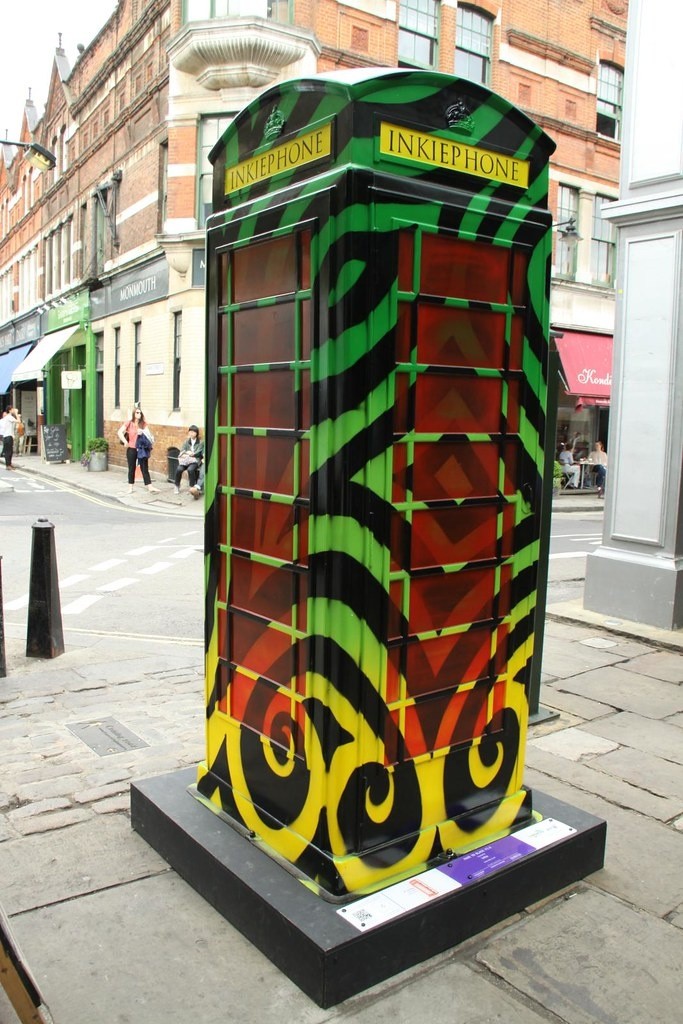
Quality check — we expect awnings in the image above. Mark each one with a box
[0,343,33,395]
[554,330,613,410]
[11,324,79,382]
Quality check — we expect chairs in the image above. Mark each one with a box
[559,459,576,491]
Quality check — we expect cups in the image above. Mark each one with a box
[580,459,583,462]
[589,459,593,463]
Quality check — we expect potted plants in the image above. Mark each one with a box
[554,461,563,499]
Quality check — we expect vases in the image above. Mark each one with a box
[88,452,108,472]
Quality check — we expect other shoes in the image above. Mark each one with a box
[190,487,199,500]
[174,486,180,494]
[5,465,15,470]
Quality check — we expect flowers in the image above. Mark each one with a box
[81,437,109,467]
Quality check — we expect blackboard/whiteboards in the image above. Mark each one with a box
[40,424,70,462]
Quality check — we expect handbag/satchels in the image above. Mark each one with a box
[120,422,131,445]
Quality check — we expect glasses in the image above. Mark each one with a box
[135,412,142,414]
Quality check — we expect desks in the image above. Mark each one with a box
[573,462,596,489]
[23,433,38,456]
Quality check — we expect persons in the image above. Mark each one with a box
[0,406,21,470]
[589,442,608,491]
[118,407,161,494]
[556,436,581,461]
[174,425,203,493]
[560,443,580,489]
[189,464,205,499]
